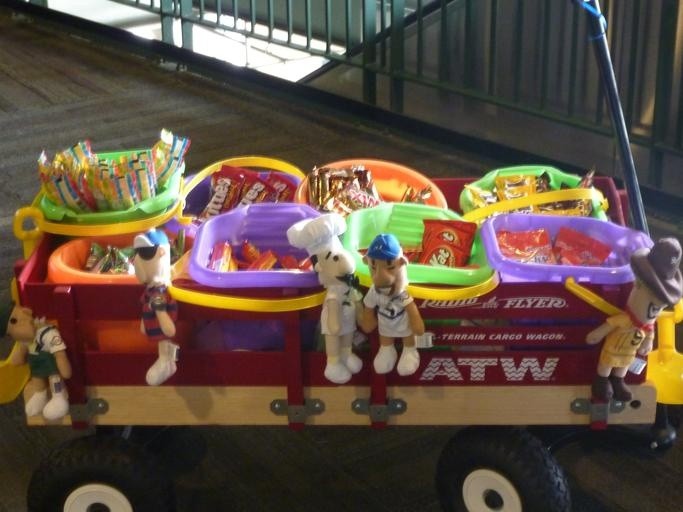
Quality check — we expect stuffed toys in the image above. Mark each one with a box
[585,236,683,403]
[9,305,73,420]
[361,235,428,382]
[130,226,186,392]
[284,213,370,384]
